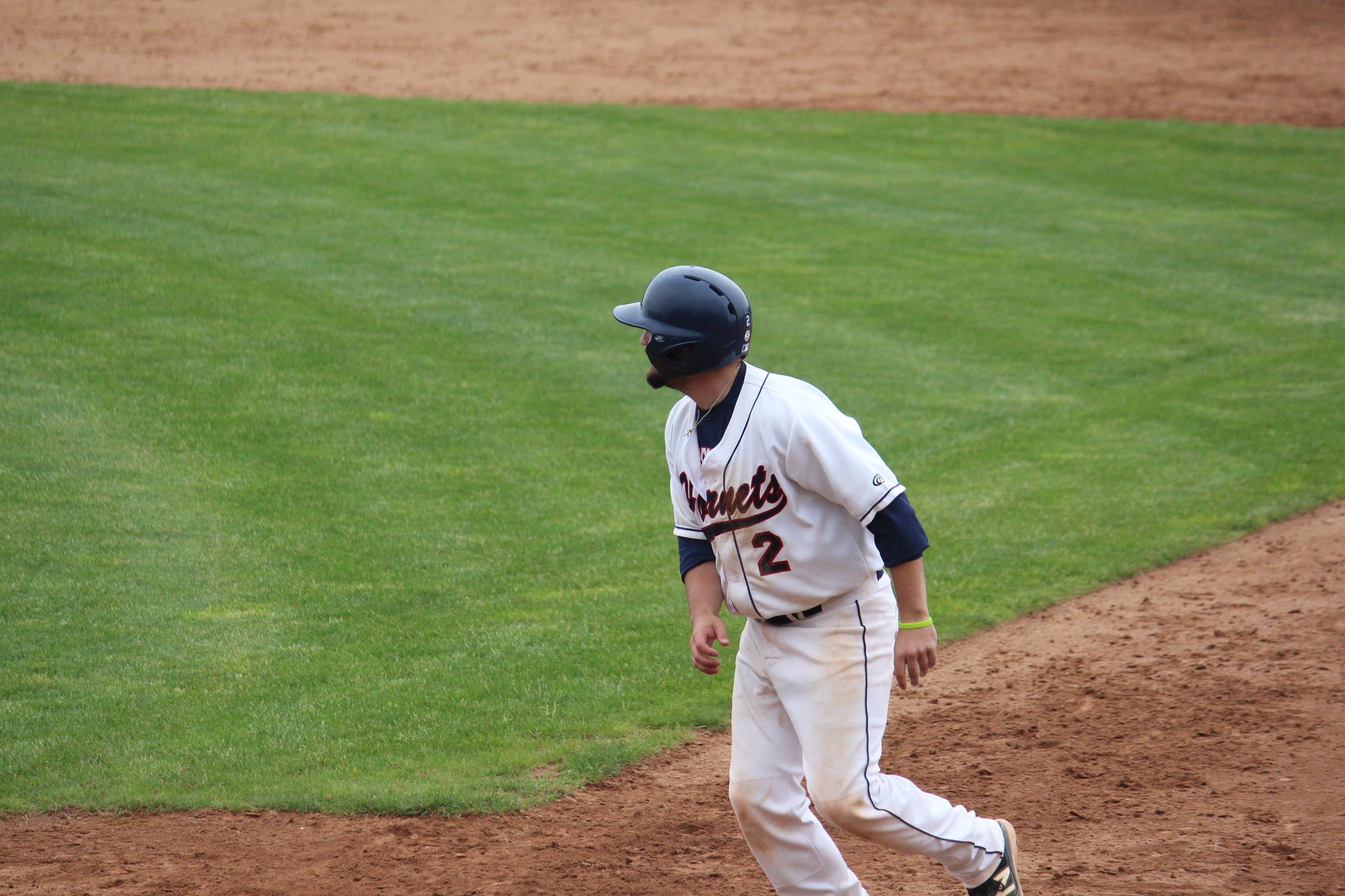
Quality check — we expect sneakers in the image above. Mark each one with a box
[967,817,1024,896]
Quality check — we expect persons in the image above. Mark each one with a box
[613,265,1024,896]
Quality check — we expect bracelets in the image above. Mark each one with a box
[898,617,933,629]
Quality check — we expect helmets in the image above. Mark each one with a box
[612,265,752,377]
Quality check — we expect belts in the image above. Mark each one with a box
[765,570,885,625]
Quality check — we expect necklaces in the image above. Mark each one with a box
[683,362,740,438]
